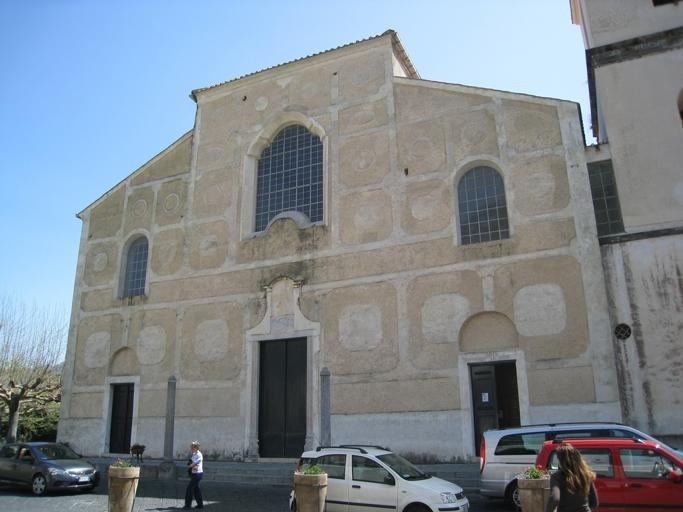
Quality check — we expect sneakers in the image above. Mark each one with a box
[183,505,203,510]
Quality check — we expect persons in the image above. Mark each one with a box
[182,440,203,510]
[547,443,599,512]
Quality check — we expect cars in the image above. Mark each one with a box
[0,442,101,497]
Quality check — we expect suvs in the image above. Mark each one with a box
[287,444,471,512]
[535,435,683,512]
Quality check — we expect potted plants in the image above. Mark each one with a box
[514,466,551,512]
[294,462,328,512]
[107,457,141,512]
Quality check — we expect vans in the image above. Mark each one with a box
[479,421,682,510]
